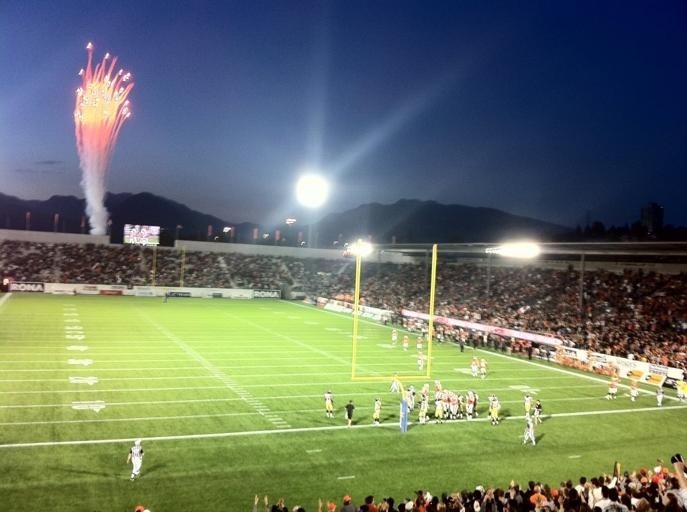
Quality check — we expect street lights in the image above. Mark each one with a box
[296,174,329,247]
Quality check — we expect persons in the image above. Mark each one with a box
[0,239,687,512]
